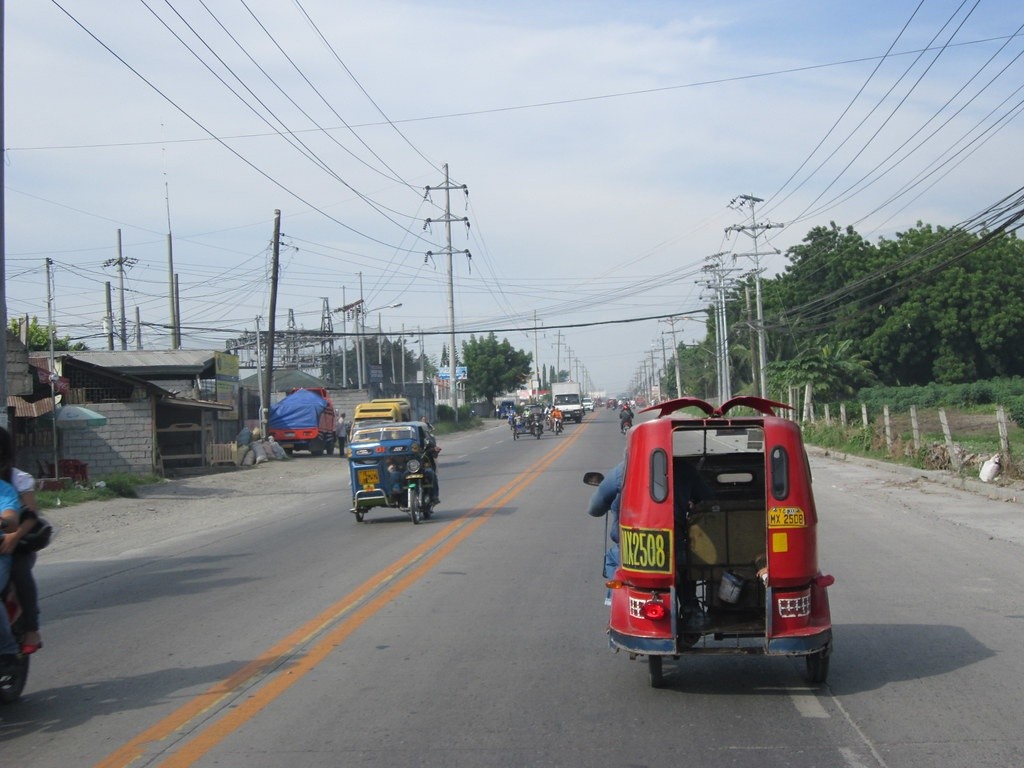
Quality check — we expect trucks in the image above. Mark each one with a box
[349,398,413,443]
[266,387,340,457]
[551,382,584,423]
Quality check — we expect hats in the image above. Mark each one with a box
[510,406,512,407]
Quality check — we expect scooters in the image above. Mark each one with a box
[620,417,634,435]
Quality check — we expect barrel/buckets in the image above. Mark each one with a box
[719,572,747,603]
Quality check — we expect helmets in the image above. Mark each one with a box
[623,404,629,409]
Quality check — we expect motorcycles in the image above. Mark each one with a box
[0,518,54,703]
[348,421,441,525]
[582,396,836,686]
[506,411,564,442]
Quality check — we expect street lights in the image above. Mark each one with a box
[362,301,402,385]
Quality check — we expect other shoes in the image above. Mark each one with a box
[340,454,347,457]
[22,633,41,654]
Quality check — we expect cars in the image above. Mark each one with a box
[583,397,594,412]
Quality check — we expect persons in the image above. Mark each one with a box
[507,400,563,435]
[588,448,706,591]
[618,397,630,408]
[0,427,41,656]
[389,417,441,511]
[335,413,347,457]
[620,406,633,428]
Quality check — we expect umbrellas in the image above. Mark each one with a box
[28,406,106,478]
